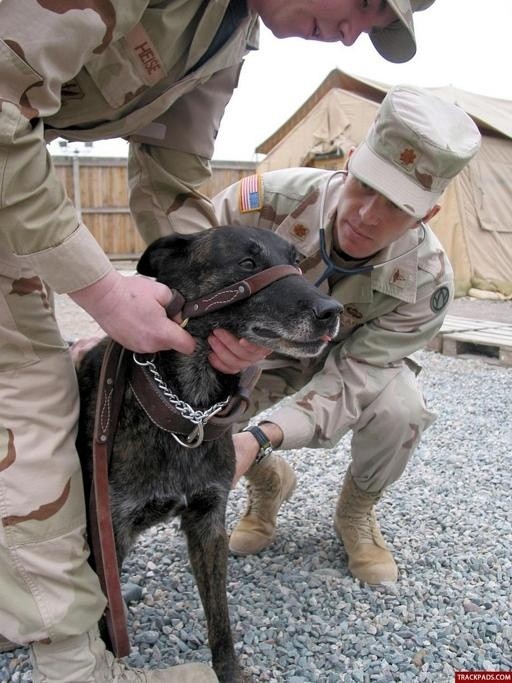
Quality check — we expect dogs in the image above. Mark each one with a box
[73,221,346,683]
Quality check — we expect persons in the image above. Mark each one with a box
[215,97,481,584]
[0,0,435,681]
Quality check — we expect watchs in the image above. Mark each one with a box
[240,426,274,465]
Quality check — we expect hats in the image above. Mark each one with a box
[348,85,482,220]
[369,0,436,63]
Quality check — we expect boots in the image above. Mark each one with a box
[336,466,399,588]
[228,453,296,556]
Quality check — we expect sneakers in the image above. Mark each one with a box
[112,658,219,683]
